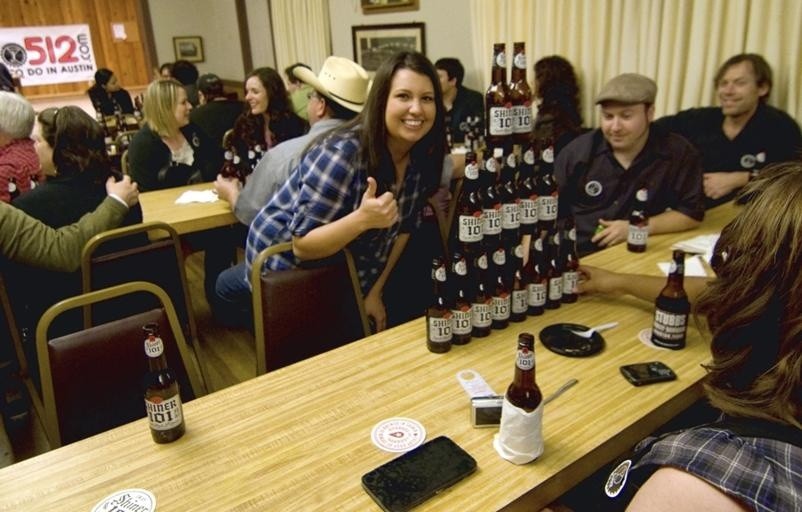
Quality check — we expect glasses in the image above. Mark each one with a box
[307,93,319,99]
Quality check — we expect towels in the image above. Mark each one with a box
[174,189,220,204]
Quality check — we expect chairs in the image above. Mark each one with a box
[102,130,142,180]
[250,240,372,378]
[77,220,214,395]
[32,279,212,452]
[221,126,234,151]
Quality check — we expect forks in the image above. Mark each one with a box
[570,321,617,339]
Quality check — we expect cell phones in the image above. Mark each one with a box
[361,435,477,512]
[620,360,677,387]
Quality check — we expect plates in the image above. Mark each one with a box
[540,323,605,358]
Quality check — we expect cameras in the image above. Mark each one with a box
[471,396,505,428]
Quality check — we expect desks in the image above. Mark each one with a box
[98,115,138,130]
[138,181,239,242]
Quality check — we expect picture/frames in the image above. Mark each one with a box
[359,0,422,16]
[171,35,206,64]
[349,20,428,81]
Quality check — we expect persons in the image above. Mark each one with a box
[3,106,155,387]
[553,73,706,259]
[246,52,451,333]
[1,175,140,277]
[652,54,801,207]
[525,56,584,156]
[435,57,484,145]
[213,53,371,302]
[620,160,801,512]
[0,45,319,202]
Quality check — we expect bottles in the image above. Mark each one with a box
[222,141,263,189]
[499,333,543,464]
[649,250,690,350]
[96,93,145,152]
[8,176,21,202]
[627,178,649,252]
[426,42,579,353]
[749,150,767,179]
[29,173,39,190]
[140,323,185,444]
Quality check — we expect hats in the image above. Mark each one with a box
[293,56,370,113]
[596,73,656,104]
